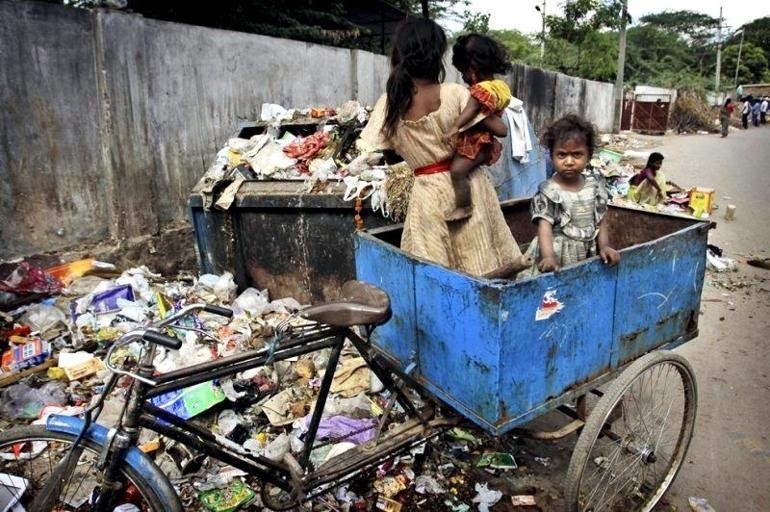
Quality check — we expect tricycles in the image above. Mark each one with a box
[0,196,717,512]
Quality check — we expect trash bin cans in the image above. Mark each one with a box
[189,96,547,310]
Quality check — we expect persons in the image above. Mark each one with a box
[627,152,665,205]
[761,98,769,124]
[719,97,740,138]
[440,33,513,223]
[359,17,523,276]
[739,97,752,129]
[735,81,744,101]
[752,98,761,127]
[515,113,622,281]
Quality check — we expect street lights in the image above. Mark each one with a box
[534,0,546,63]
[732,25,745,84]
